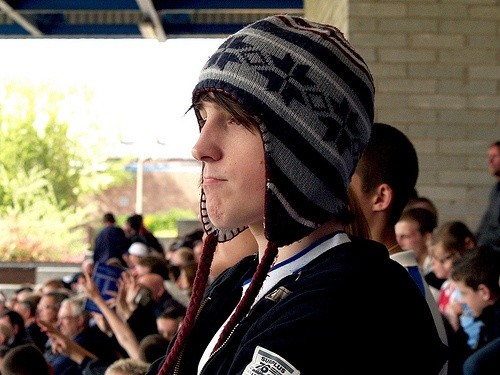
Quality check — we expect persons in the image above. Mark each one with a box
[147,14,448,375]
[350,122,500,375]
[0,213,259,375]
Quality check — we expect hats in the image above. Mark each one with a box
[156,13,374,375]
[129,242,149,258]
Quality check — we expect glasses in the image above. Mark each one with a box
[432,252,456,264]
